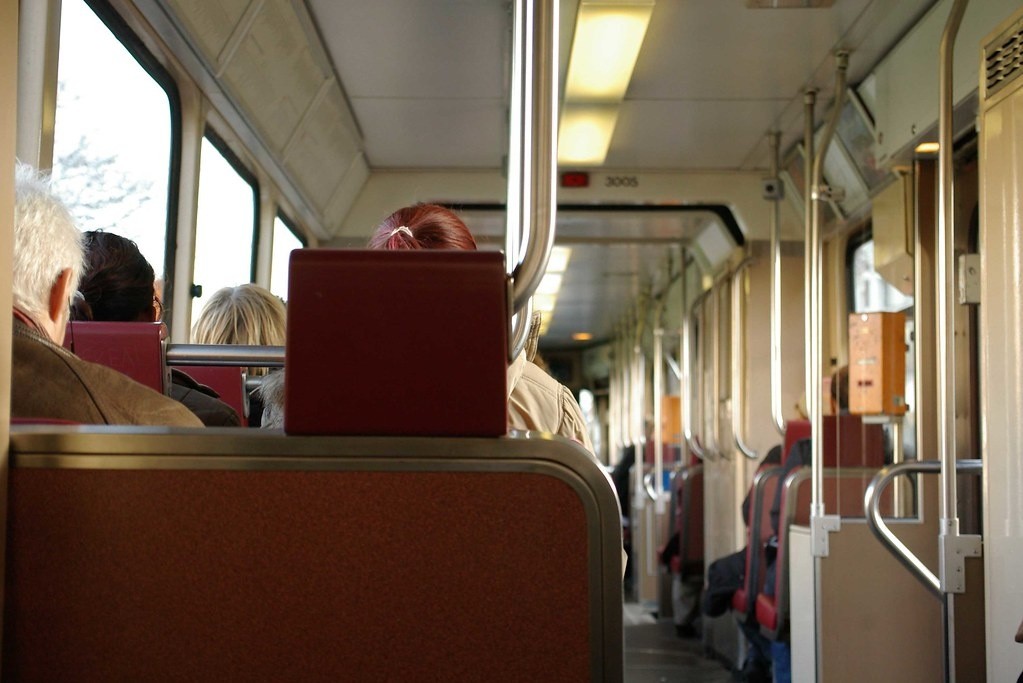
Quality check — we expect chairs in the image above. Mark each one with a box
[756,415,894,644]
[626,432,704,583]
[729,420,811,625]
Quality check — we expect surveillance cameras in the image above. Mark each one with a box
[762,179,784,198]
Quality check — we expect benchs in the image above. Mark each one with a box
[0,249,623,683]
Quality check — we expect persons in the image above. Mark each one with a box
[10,157,241,429]
[701,367,853,683]
[251,201,597,451]
[187,284,286,375]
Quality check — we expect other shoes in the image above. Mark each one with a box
[676,623,701,640]
[738,650,772,683]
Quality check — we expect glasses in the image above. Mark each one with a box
[152,296,164,321]
